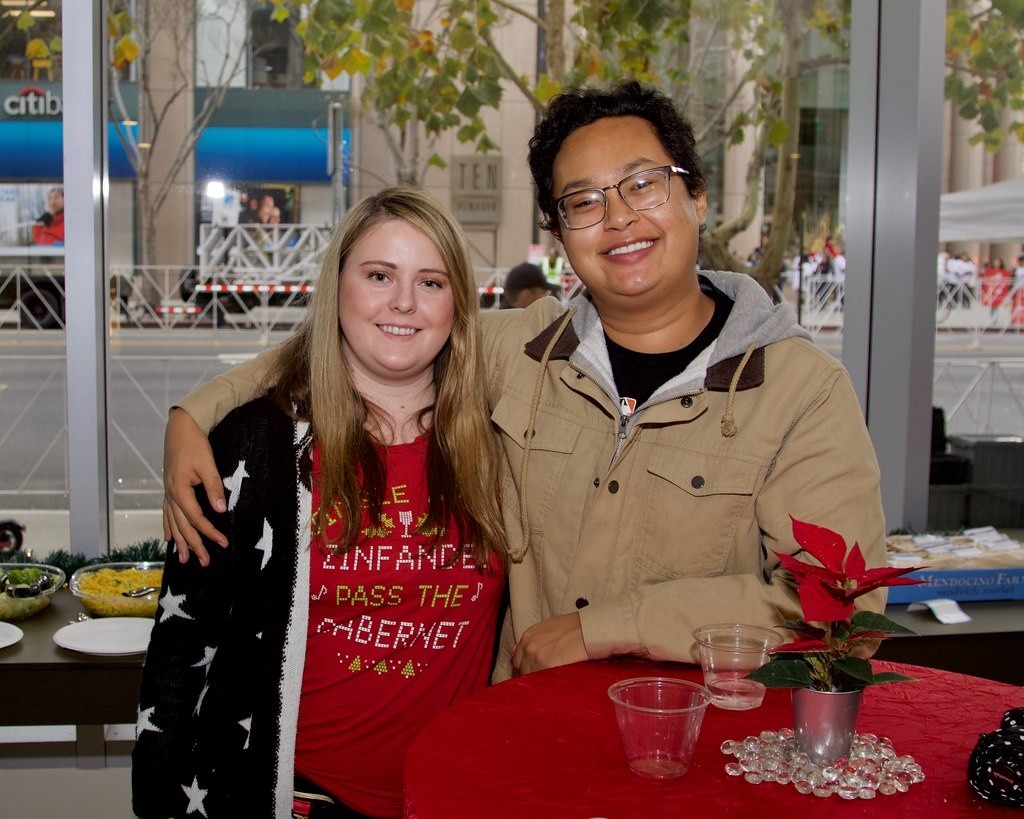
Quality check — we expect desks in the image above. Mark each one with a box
[0,589,154,727]
[405,656,1024,819]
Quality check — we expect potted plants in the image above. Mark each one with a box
[739,512,936,762]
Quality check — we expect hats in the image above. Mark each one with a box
[504,263,561,292]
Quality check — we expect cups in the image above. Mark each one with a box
[691,623,784,710]
[608,676,714,780]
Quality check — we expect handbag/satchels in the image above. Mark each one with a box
[968,707,1024,808]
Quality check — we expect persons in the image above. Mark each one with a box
[936,251,1024,325]
[223,188,280,247]
[748,234,846,308]
[540,248,565,290]
[133,186,508,819]
[32,187,64,245]
[494,262,561,309]
[162,78,891,686]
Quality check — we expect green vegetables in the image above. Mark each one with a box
[5,565,45,587]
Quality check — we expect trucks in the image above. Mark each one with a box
[0,120,351,331]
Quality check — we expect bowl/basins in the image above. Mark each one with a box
[0,562,66,621]
[68,560,164,616]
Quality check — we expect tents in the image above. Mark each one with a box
[938,171,1024,281]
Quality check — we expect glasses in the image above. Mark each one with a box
[551,165,690,231]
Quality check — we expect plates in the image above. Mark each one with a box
[52,616,156,656]
[0,621,24,649]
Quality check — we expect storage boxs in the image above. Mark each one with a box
[884,531,1024,604]
